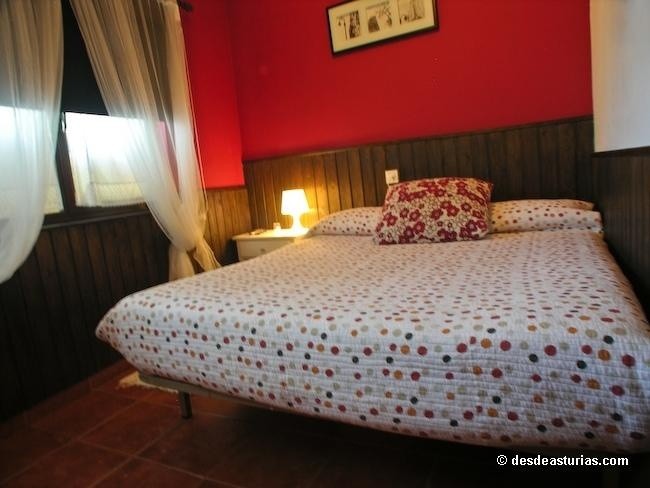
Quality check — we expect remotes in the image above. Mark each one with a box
[250,229,265,235]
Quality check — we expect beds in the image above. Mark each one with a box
[96,199,650,486]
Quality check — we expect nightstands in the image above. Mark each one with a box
[231,226,309,262]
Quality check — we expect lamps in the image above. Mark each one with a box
[280,190,310,231]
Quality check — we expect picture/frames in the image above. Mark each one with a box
[324,0,440,55]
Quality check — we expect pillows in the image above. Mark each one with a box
[375,177,494,243]
